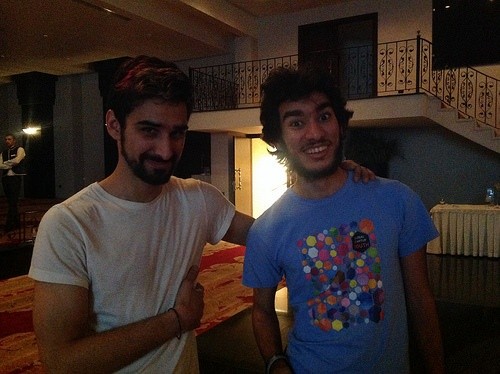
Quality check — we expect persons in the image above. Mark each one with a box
[0,133,27,234]
[27,55,374,374]
[241,66,445,374]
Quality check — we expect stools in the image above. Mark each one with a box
[18,201,41,243]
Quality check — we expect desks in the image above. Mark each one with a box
[426,203,500,257]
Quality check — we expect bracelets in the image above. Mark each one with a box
[266,353,287,374]
[169,308,182,341]
